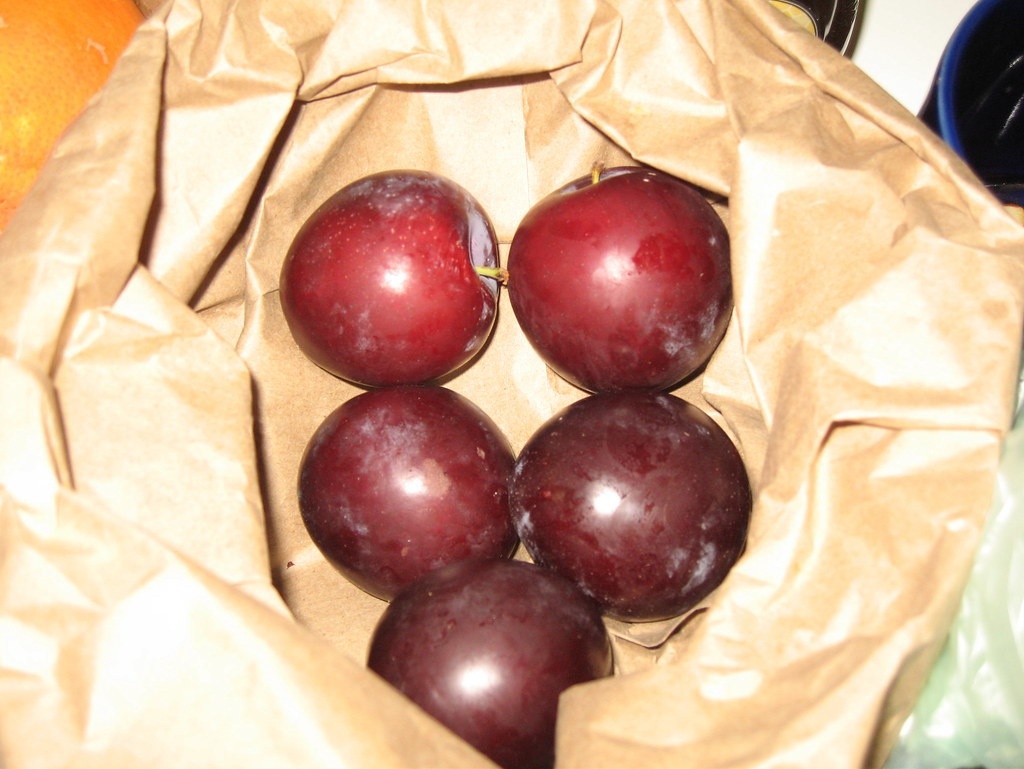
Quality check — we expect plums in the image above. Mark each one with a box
[279,157,756,769]
